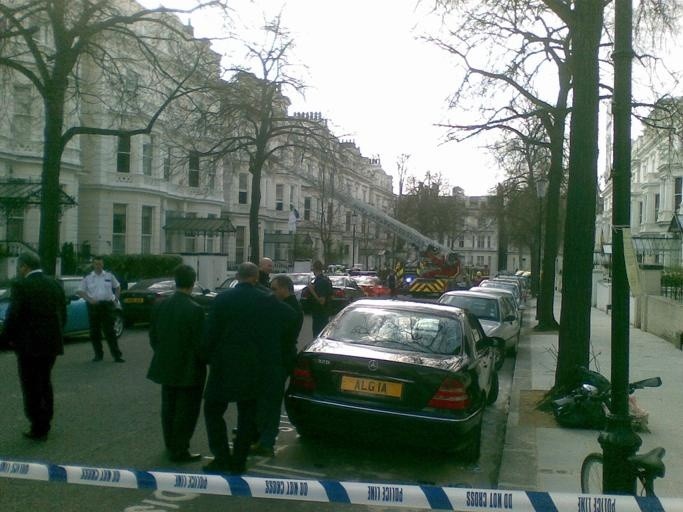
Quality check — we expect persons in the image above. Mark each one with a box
[307,261,332,338]
[192,261,277,477]
[147,263,207,464]
[389,271,396,296]
[1,251,66,441]
[257,258,273,288]
[247,274,302,453]
[76,255,127,362]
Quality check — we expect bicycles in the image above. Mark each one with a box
[580,445,666,496]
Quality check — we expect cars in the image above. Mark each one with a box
[281,299,505,464]
[214,277,269,293]
[468,287,519,319]
[493,270,543,300]
[267,272,291,282]
[299,276,364,309]
[287,272,315,304]
[435,290,520,367]
[322,262,390,298]
[0,277,123,345]
[478,279,520,309]
[118,278,218,327]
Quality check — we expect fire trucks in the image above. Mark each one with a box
[262,151,465,299]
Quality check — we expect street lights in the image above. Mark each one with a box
[350,209,357,267]
